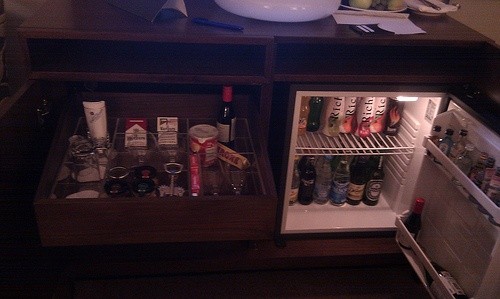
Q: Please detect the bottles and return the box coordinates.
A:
[397,198,424,250]
[421,252,470,299]
[290,155,300,205]
[306,96,323,132]
[216,86,237,149]
[447,129,468,163]
[313,155,332,205]
[362,155,384,206]
[298,96,309,132]
[432,129,454,164]
[427,125,442,157]
[298,156,316,205]
[346,155,367,205]
[452,144,474,186]
[464,152,500,230]
[331,156,350,206]
[321,95,404,137]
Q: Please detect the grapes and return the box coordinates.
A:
[370,0,388,11]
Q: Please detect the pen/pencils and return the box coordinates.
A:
[192,17,244,30]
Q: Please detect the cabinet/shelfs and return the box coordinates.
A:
[0,0,500,299]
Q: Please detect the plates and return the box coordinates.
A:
[339,0,451,17]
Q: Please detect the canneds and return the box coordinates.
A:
[189,124,218,167]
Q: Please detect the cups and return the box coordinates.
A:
[70,133,188,197]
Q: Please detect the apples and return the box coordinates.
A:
[349,0,371,9]
[387,0,403,11]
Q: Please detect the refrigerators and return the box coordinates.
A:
[275,79,500,299]
